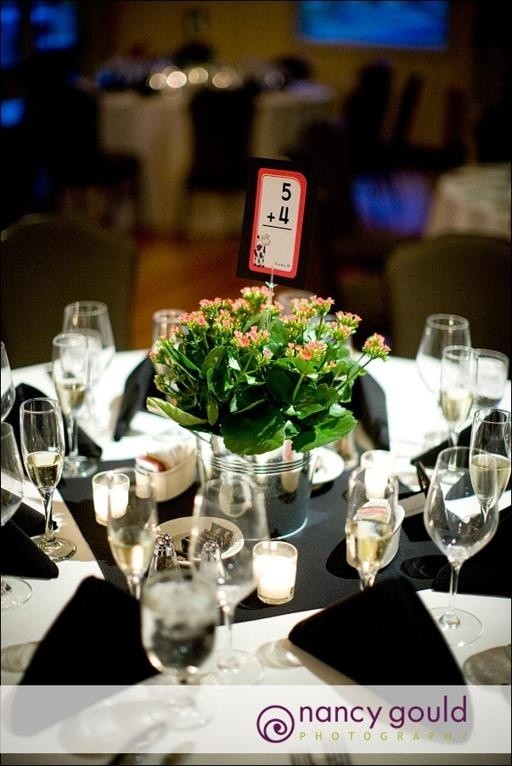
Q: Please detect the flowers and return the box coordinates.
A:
[145,286,391,457]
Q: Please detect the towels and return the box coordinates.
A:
[115,354,166,446]
[411,408,512,470]
[8,575,162,734]
[2,382,104,459]
[287,574,472,743]
[341,362,389,451]
[0,488,60,577]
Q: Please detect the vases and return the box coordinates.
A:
[196,431,315,542]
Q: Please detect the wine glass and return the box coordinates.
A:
[109,465,159,605]
[346,464,405,591]
[417,445,501,651]
[416,311,512,530]
[141,535,218,729]
[187,476,271,687]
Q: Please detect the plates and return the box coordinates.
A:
[157,516,244,568]
[242,440,345,493]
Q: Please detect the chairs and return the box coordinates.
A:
[386,230,511,374]
[42,40,512,244]
[0,211,138,369]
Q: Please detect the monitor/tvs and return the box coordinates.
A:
[296,1,448,56]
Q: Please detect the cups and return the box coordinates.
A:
[253,540,298,607]
[89,471,129,524]
[153,306,187,348]
